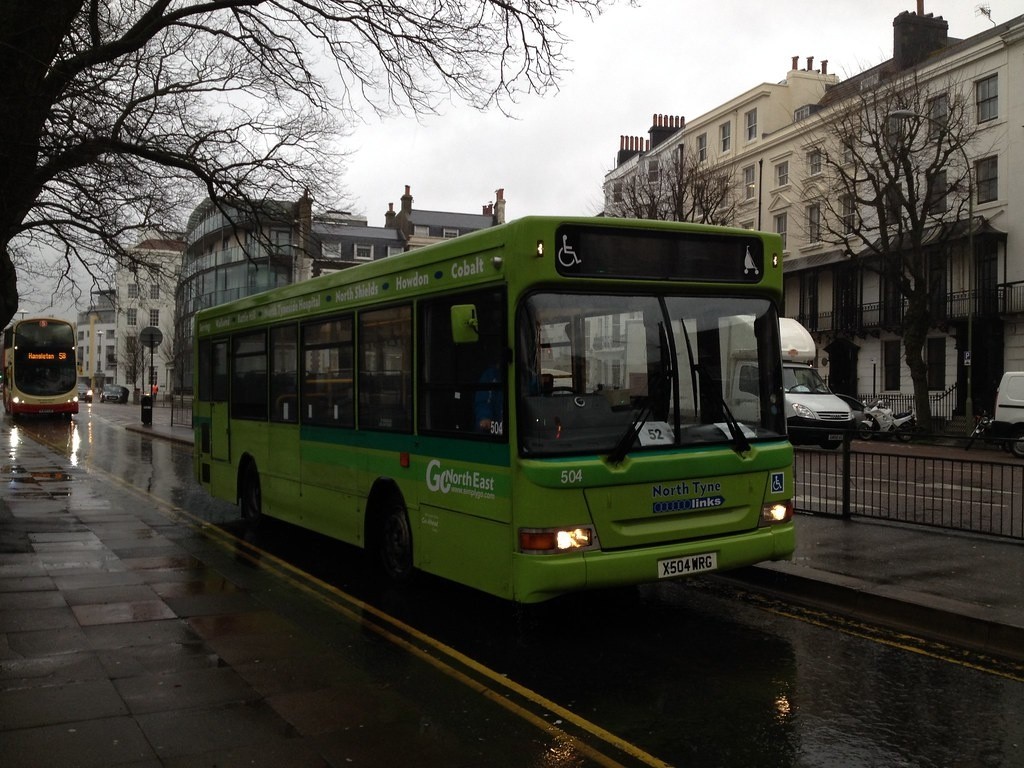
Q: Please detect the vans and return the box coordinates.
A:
[994,371,1024,453]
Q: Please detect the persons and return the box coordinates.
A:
[473,339,544,432]
[152,381,159,402]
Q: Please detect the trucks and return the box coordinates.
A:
[620,314,858,451]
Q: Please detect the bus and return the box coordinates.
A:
[189,215,795,606]
[2,317,79,423]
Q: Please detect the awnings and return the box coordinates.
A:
[783,215,1008,275]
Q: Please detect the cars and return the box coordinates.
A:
[78,382,93,403]
[100,384,129,403]
[835,393,865,429]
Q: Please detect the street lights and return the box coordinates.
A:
[890,110,972,397]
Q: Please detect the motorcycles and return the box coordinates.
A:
[858,401,918,442]
[964,408,1024,459]
